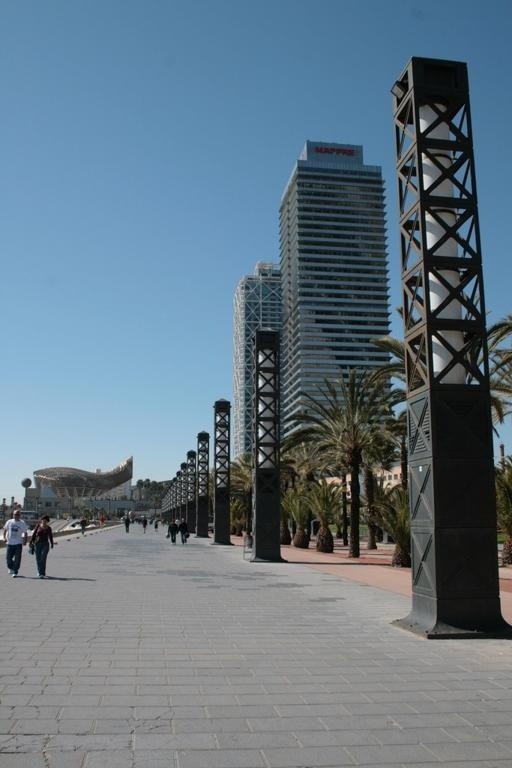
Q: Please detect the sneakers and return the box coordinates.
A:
[8,569,17,577]
[40,575,44,579]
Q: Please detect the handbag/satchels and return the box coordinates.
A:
[166,534,169,538]
[28,543,34,554]
[185,534,189,538]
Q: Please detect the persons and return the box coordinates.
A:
[178,518,188,545]
[142,518,147,534]
[81,516,87,534]
[168,519,179,544]
[29,514,55,579]
[125,517,131,533]
[3,509,29,578]
